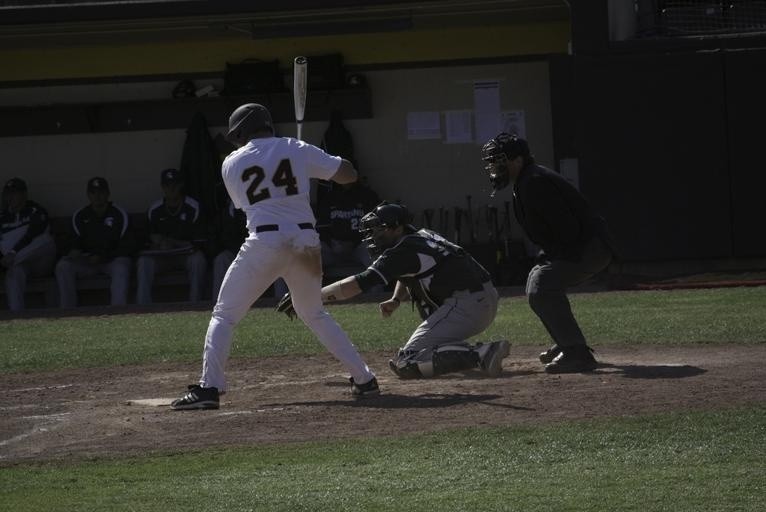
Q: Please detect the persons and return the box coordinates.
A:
[135,167,210,304]
[53,176,130,308]
[0,177,45,311]
[211,195,286,308]
[311,160,382,294]
[276,201,514,379]
[166,102,384,411]
[479,133,616,375]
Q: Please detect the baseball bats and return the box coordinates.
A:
[293,57,307,140]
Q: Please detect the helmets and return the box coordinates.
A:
[479,132,529,194]
[222,102,277,146]
[357,197,412,260]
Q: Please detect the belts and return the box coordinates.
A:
[255,223,314,234]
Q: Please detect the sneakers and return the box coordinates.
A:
[538,343,601,374]
[346,376,382,396]
[479,337,511,378]
[168,383,220,411]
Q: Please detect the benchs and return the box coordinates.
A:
[0,213,201,308]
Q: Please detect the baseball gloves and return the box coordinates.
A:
[274,293,297,318]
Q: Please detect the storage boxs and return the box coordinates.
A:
[302,50,346,91]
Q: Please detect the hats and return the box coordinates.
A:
[2,177,28,193]
[161,168,189,184]
[86,175,109,192]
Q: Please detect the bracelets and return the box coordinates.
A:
[392,297,400,307]
